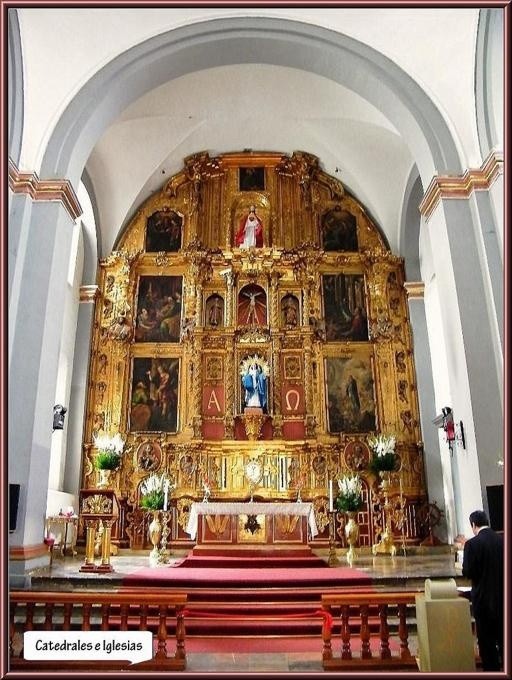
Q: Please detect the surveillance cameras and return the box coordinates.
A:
[431,412,451,426]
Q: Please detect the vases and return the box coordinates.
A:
[373,471,400,555]
[97,467,111,489]
[149,510,162,559]
[345,512,359,560]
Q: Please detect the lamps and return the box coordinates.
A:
[53,404,67,429]
[446,420,466,453]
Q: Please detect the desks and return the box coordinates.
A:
[46,516,79,557]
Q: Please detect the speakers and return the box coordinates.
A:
[487,484,504,534]
[8,483,20,534]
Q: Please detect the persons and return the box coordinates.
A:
[341,307,368,341]
[138,445,159,471]
[462,510,504,672]
[326,204,354,236]
[146,364,177,422]
[237,206,262,249]
[209,298,221,324]
[345,375,360,409]
[242,363,268,409]
[243,290,262,324]
[346,443,367,470]
[282,297,296,324]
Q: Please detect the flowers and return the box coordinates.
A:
[367,434,397,473]
[140,476,168,509]
[92,433,125,469]
[337,476,364,512]
